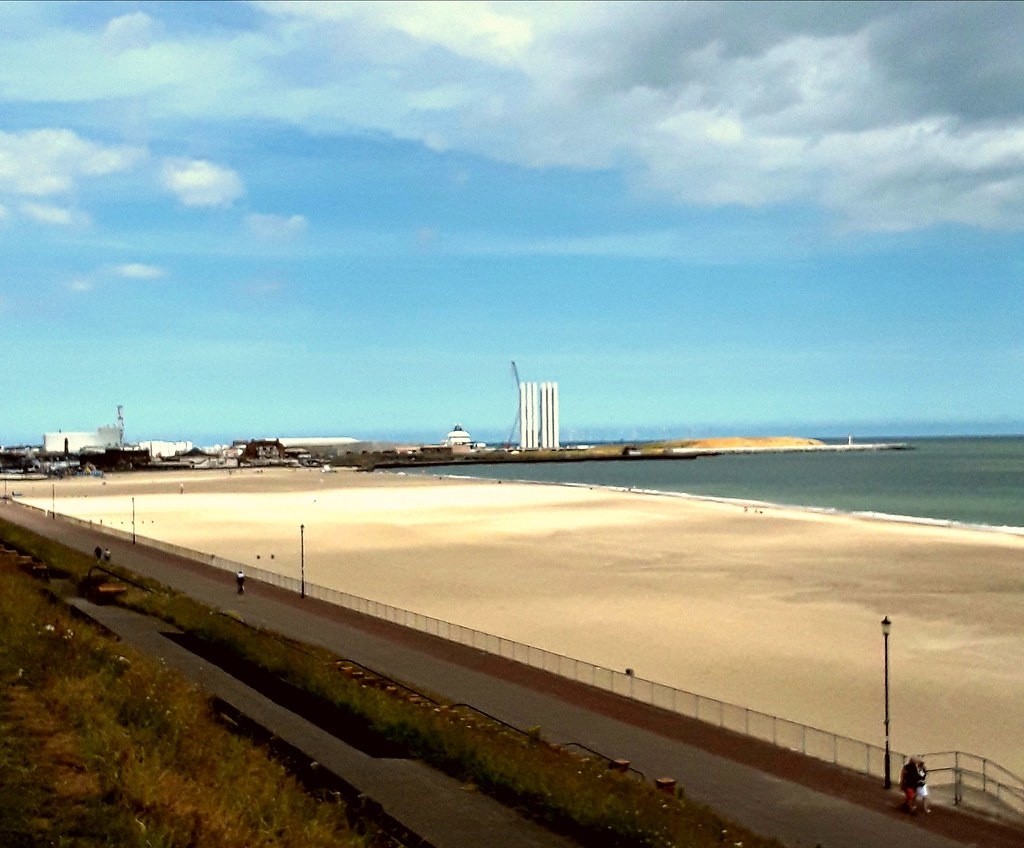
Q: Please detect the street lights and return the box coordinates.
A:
[300,524,305,598]
[53,484,54,519]
[881,615,893,790]
[132,497,135,544]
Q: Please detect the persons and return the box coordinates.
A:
[236,571,245,594]
[94,545,102,559]
[915,760,932,814]
[898,755,920,815]
[104,548,110,560]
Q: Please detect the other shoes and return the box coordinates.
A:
[927,810,932,815]
